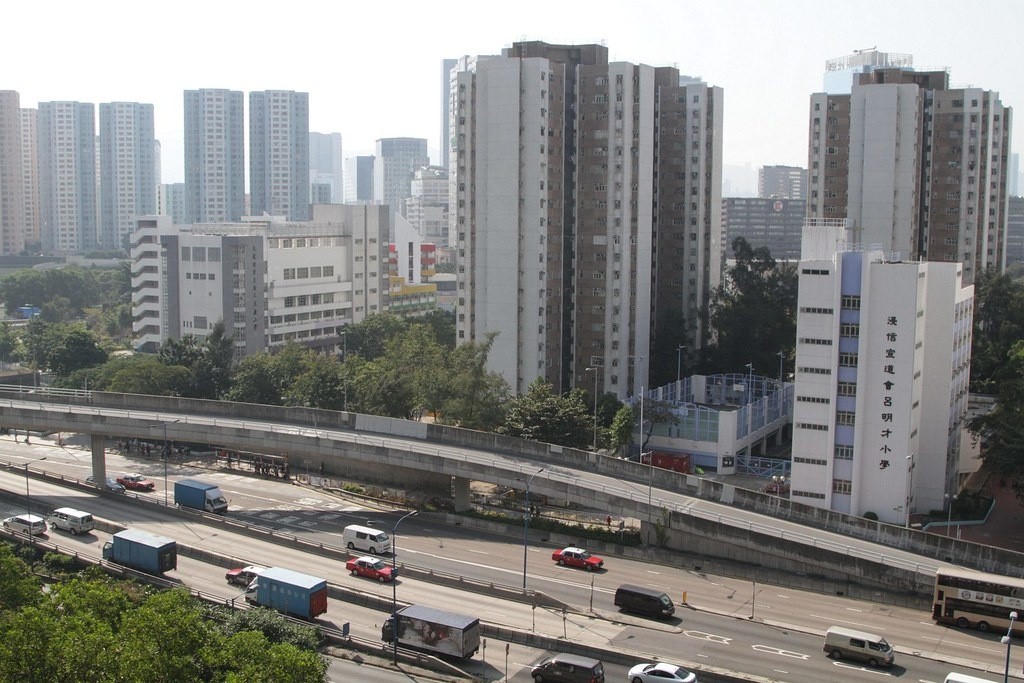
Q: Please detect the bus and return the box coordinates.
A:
[931,566,1024,636]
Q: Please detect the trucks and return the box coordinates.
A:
[381,603,480,662]
[173,479,232,515]
[103,528,177,576]
[245,566,328,621]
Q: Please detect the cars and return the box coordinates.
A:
[628,663,698,683]
[86,473,155,494]
[552,547,604,571]
[346,555,394,582]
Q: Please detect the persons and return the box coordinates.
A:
[529,505,540,517]
[117,439,192,461]
[481,494,487,511]
[220,451,291,480]
[619,521,626,531]
[321,462,325,477]
[423,623,436,646]
[606,515,612,528]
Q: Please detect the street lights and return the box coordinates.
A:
[340,331,347,412]
[945,494,957,536]
[745,364,757,466]
[772,475,786,495]
[777,352,785,417]
[586,367,598,451]
[26,303,37,396]
[676,345,686,401]
[6,456,47,573]
[150,419,180,507]
[367,510,419,664]
[515,468,545,594]
[906,455,913,527]
[642,452,652,545]
[1001,611,1018,683]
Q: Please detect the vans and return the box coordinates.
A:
[3,507,94,536]
[614,583,675,618]
[343,524,390,554]
[823,626,894,666]
[530,652,605,683]
[944,672,998,683]
